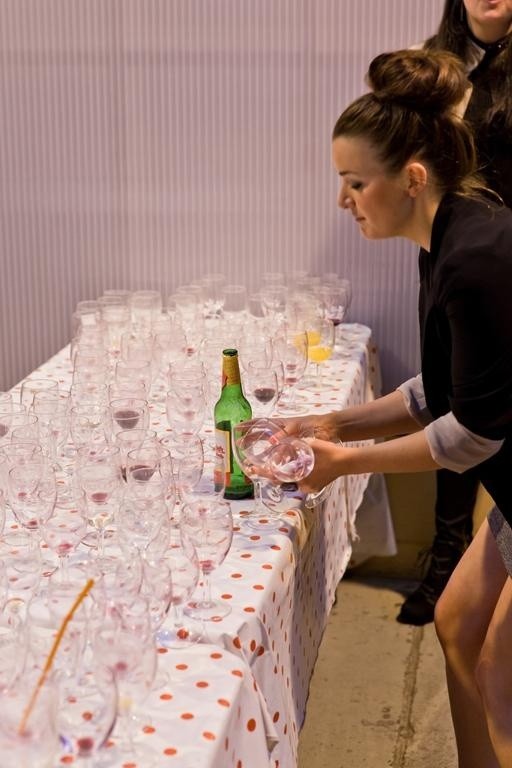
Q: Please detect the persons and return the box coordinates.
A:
[236,46,511,768]
[395,1,511,627]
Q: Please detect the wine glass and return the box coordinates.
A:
[0,263,352,768]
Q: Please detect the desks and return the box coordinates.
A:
[0,320,399,768]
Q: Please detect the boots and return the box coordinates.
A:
[396,493,478,626]
